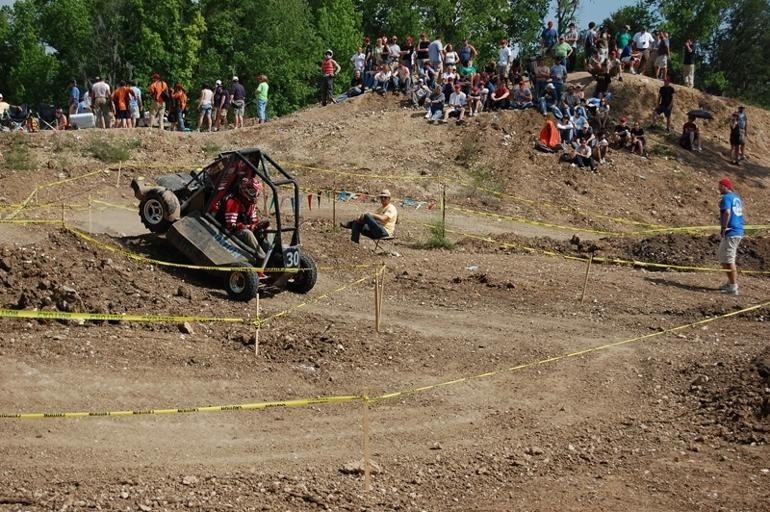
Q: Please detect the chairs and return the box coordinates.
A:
[373,216,401,257]
[4,102,32,131]
[36,103,58,132]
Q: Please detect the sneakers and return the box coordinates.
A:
[455,119,465,126]
[438,118,449,124]
[721,284,739,295]
[429,118,436,125]
[203,128,212,132]
[468,111,473,117]
[473,111,479,118]
[194,128,202,132]
[340,220,353,229]
[718,282,734,290]
[425,111,433,119]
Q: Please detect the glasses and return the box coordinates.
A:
[379,195,389,199]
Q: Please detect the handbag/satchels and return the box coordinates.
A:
[161,90,172,103]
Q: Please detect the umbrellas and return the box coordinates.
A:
[686,108,713,129]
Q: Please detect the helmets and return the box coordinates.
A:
[236,177,263,204]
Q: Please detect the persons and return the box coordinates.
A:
[252,75,269,125]
[229,76,246,128]
[149,72,168,130]
[730,113,740,165]
[321,49,341,106]
[717,177,743,296]
[196,79,230,132]
[341,188,397,244]
[736,104,747,161]
[170,84,190,131]
[350,21,676,171]
[228,179,271,258]
[683,36,696,89]
[0,75,143,131]
[680,116,702,152]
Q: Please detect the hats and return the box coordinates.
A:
[500,38,508,44]
[576,108,583,114]
[215,79,222,86]
[563,114,570,118]
[150,73,161,80]
[375,189,392,197]
[546,83,556,91]
[231,75,240,82]
[717,176,735,193]
[325,49,334,55]
[453,83,462,89]
[471,86,481,93]
[620,115,629,123]
[587,126,594,133]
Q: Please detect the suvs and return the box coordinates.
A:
[131,148,317,302]
